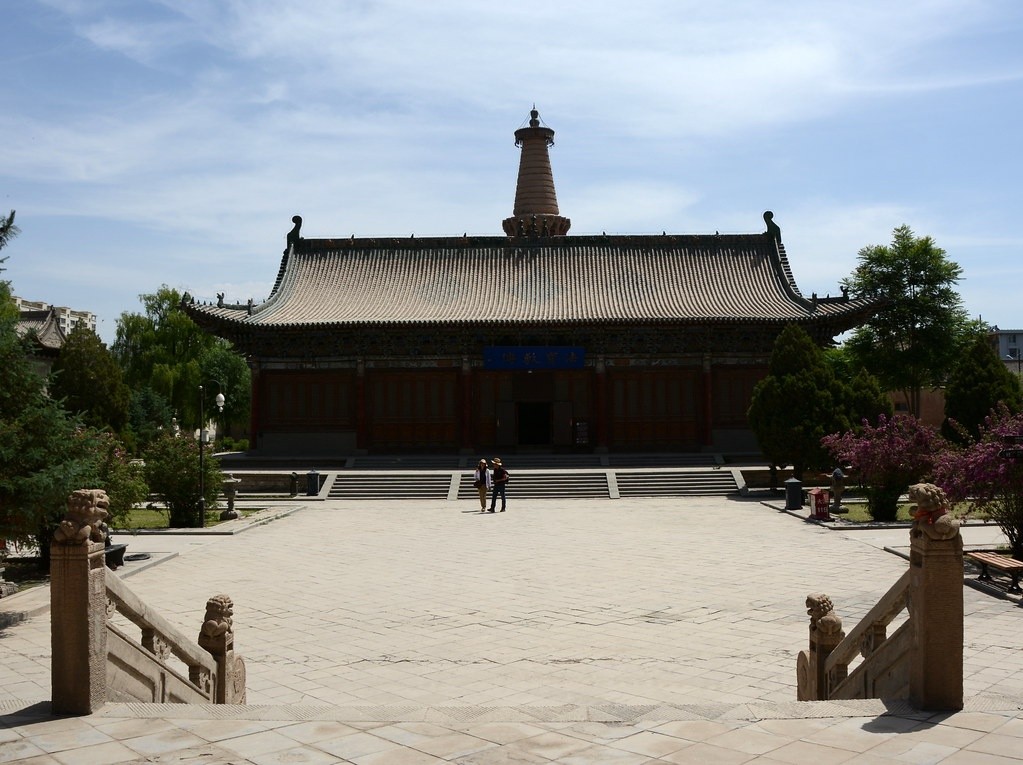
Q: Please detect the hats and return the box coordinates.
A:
[491,458,502,465]
[479,459,488,467]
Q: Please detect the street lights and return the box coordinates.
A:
[198,378,225,528]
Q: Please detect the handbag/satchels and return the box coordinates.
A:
[505,471,509,483]
[474,480,484,488]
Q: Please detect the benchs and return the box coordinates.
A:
[105,544,129,571]
[967,552,1023,594]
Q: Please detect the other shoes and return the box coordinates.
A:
[488,508,495,513]
[500,508,505,512]
[481,507,485,511]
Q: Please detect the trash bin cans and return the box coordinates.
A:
[307,468,319,496]
[784,474,803,510]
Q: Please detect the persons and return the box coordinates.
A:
[486,458,507,512]
[473,459,490,512]
